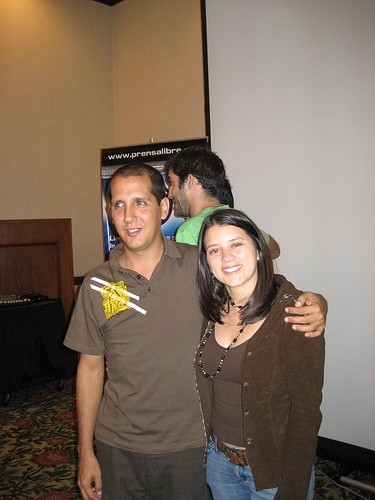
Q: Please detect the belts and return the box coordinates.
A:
[217,440,248,467]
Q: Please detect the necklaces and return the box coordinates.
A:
[198,296,247,377]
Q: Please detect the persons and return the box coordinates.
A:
[194,209,325,500]
[64,162,328,500]
[163,149,280,260]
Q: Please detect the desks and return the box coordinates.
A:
[0,295,78,406]
